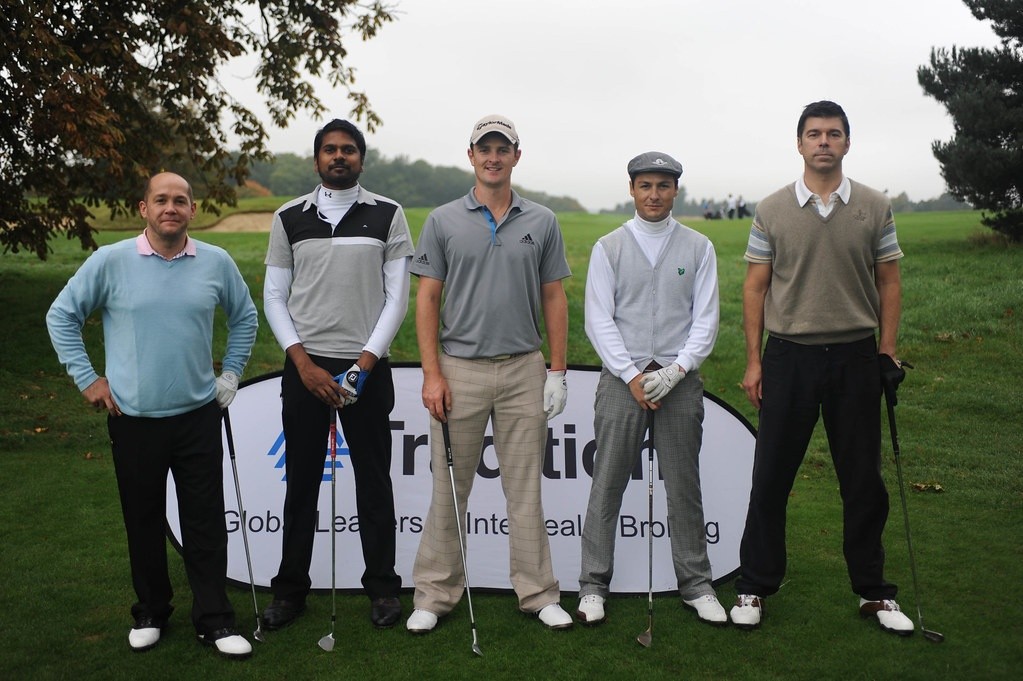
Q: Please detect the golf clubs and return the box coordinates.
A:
[224,403,267,645]
[883,385,945,643]
[317,404,339,653]
[439,399,485,657]
[636,408,657,649]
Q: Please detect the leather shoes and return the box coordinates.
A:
[731,592,763,626]
[199,624,252,656]
[128,616,163,649]
[576,594,606,624]
[532,602,573,628]
[408,609,438,635]
[859,595,914,635]
[682,590,727,625]
[371,593,400,628]
[262,593,306,630]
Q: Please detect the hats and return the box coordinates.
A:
[469,116,521,152]
[628,153,682,181]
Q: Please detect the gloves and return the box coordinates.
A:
[872,351,907,406]
[332,363,370,408]
[214,367,239,410]
[638,363,685,403]
[543,367,571,421]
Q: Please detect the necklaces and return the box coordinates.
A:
[501,200,513,216]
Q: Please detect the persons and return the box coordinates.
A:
[46,172,257,656]
[701,194,751,220]
[257,119,404,628]
[575,151,729,625]
[406,114,572,633]
[730,101,915,635]
